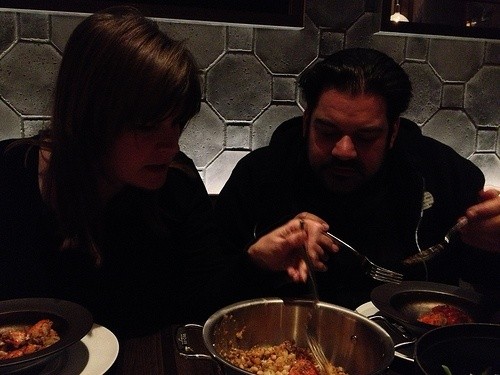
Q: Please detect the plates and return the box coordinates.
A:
[9,323,119,375]
[355,301,415,362]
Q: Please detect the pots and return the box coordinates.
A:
[168,297,417,375]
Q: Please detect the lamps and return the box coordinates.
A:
[390,0,409,23]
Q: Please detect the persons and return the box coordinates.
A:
[216,48,500,307]
[0,5,324,323]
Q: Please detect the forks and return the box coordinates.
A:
[325,231,403,284]
[405,215,468,263]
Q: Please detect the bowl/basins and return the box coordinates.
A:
[415,323,500,375]
[370,281,494,333]
[0,299,94,375]
